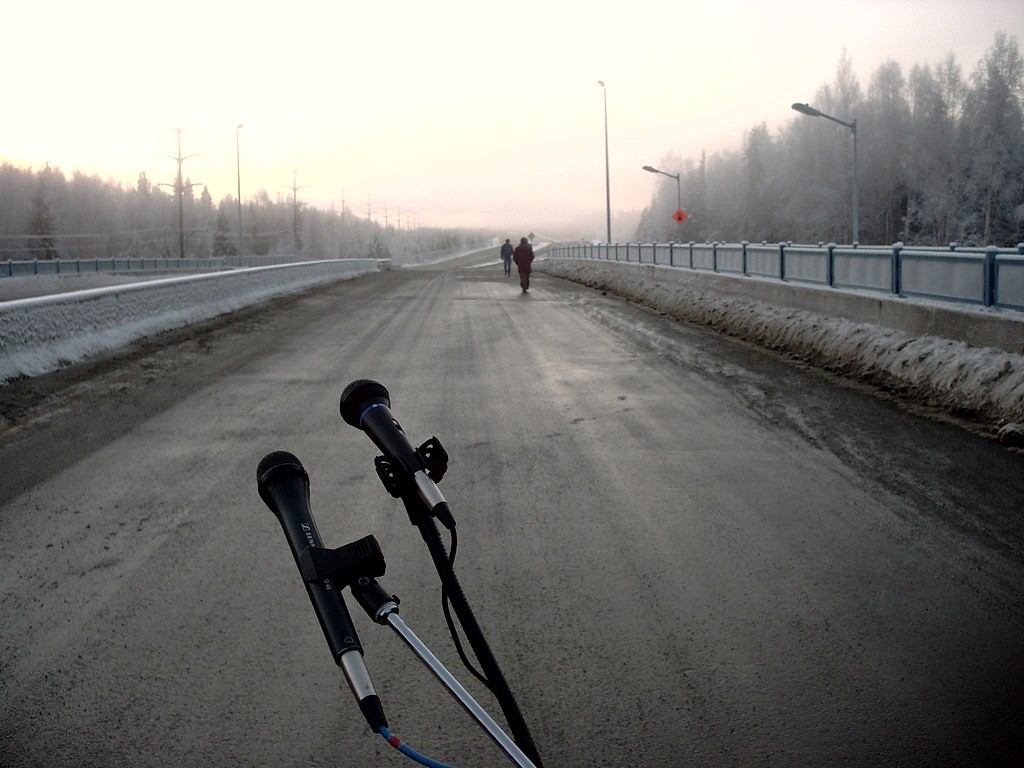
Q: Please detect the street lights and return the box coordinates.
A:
[641,165,683,242]
[598,80,613,243]
[792,101,860,245]
[236,124,244,265]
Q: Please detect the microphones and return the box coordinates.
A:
[258,450,388,733]
[341,379,457,531]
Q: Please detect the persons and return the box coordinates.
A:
[513,238,535,295]
[501,239,514,277]
